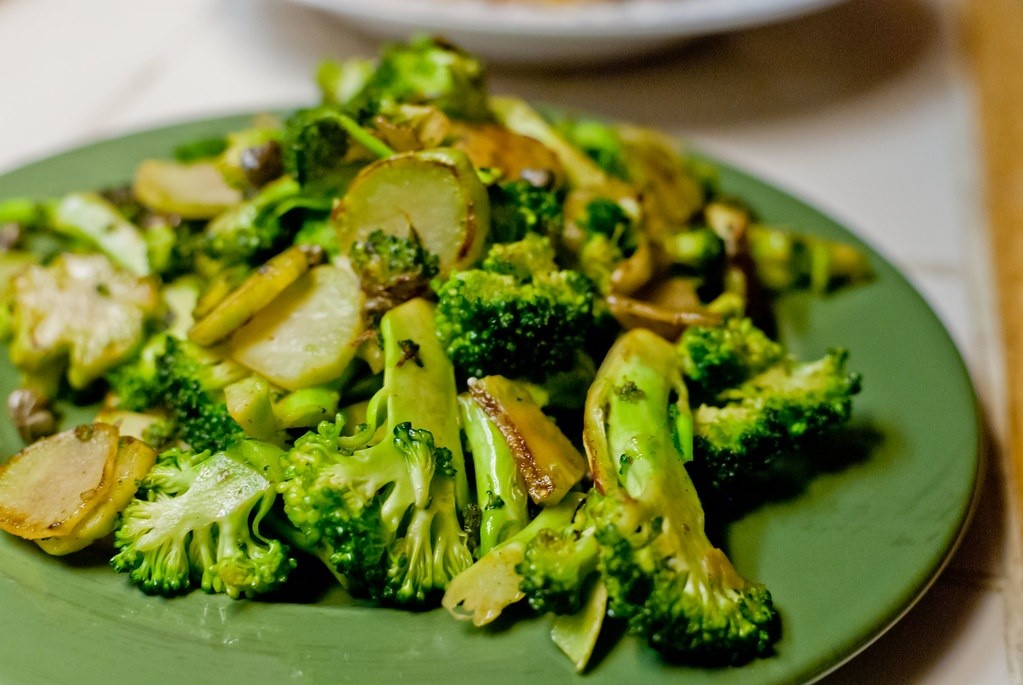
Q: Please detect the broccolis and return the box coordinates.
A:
[2,34,863,662]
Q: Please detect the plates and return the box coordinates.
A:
[1,106,982,685]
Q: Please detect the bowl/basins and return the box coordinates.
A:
[301,1,844,68]
